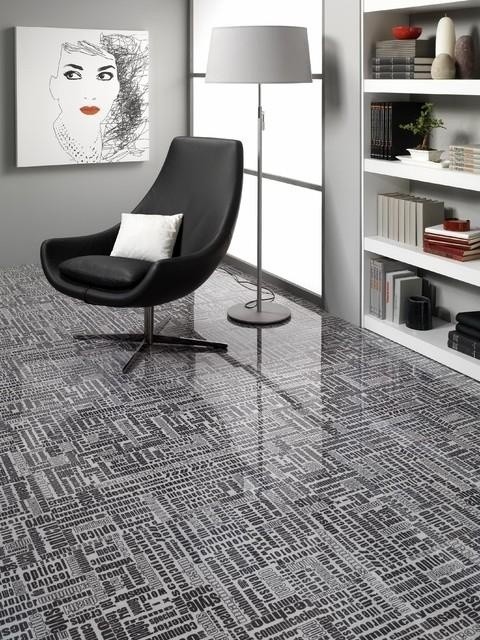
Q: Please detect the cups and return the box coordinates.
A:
[407,294,434,331]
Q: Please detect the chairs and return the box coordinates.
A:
[40,133,247,375]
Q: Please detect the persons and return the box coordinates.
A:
[49,33,145,164]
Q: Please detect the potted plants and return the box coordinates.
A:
[397,101,451,164]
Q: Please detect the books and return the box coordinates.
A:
[370,36,436,80]
[370,100,479,175]
[366,191,479,360]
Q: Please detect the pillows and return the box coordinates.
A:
[102,211,185,263]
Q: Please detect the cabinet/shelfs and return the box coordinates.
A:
[359,3,480,385]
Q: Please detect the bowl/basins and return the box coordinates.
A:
[391,27,422,39]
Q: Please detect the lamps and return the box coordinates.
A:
[203,23,317,329]
